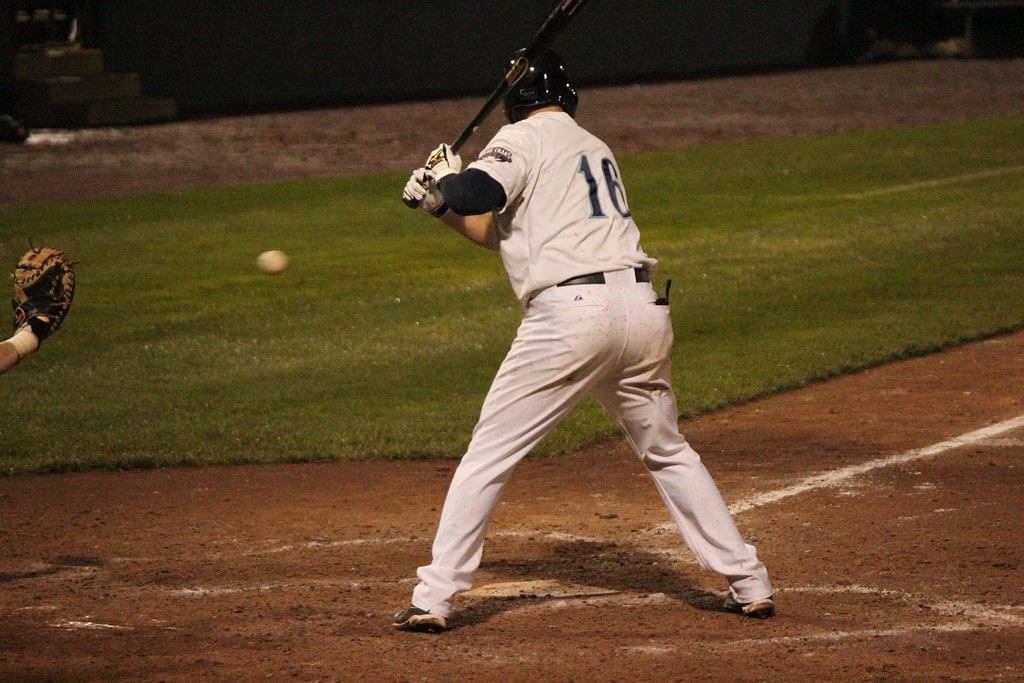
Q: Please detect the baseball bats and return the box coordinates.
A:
[402,0,590,209]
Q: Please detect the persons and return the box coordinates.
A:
[389,45,777,634]
[0,314,50,374]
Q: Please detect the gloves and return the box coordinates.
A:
[402,168,450,220]
[424,143,462,181]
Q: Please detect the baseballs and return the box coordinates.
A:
[256,249,288,273]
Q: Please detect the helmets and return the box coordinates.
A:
[502,48,578,121]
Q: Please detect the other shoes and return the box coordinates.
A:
[393,605,448,629]
[726,596,774,615]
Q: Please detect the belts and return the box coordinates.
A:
[557,268,650,286]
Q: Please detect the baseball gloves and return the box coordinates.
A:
[12,247,76,344]
